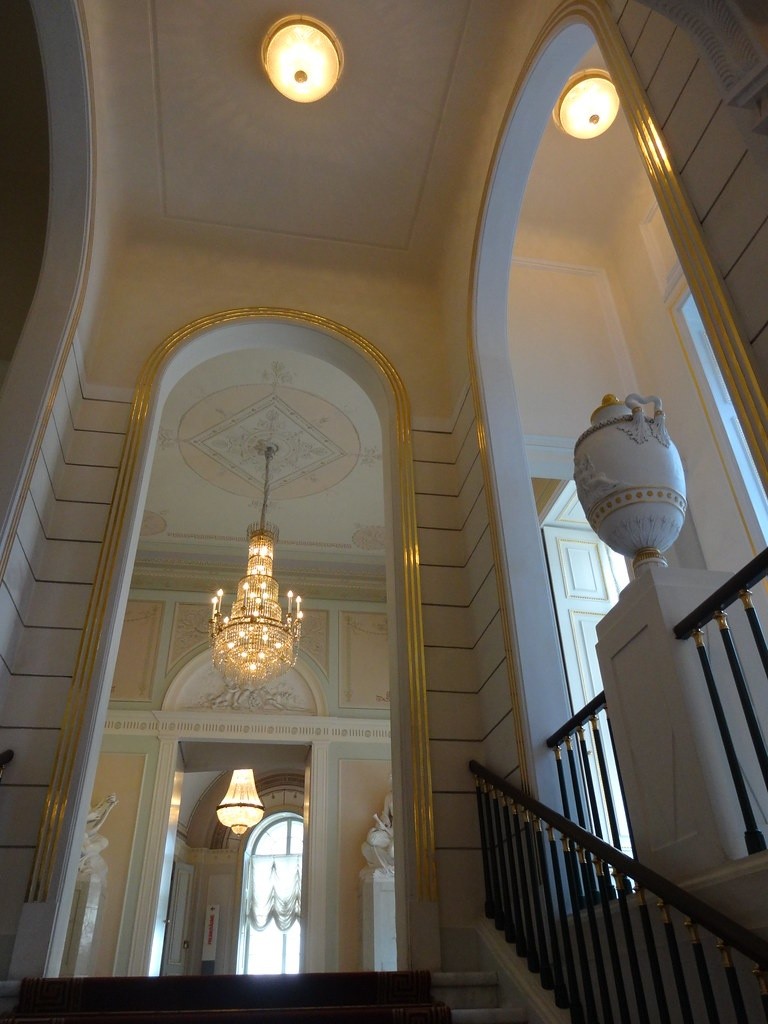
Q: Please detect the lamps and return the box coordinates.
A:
[216,769,266,837]
[209,439,305,680]
[553,67,620,139]
[261,13,345,103]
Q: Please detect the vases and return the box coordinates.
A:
[573,392,689,575]
